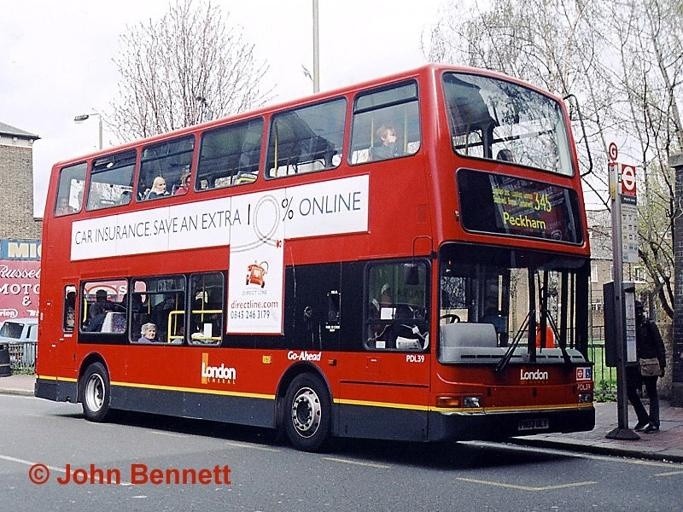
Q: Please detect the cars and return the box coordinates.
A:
[0,318,37,367]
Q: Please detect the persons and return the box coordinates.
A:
[626,297,667,432]
[88,290,115,320]
[174,173,191,195]
[365,123,407,163]
[137,321,158,343]
[58,196,74,214]
[66,291,75,327]
[145,176,169,199]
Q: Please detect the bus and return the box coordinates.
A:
[30,65,595,456]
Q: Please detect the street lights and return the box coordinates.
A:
[71,111,103,151]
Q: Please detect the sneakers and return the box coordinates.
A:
[634,421,648,429]
[645,425,658,434]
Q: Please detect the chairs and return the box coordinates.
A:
[98,199,116,208]
[234,168,259,185]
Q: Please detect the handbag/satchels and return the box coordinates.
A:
[639,358,662,376]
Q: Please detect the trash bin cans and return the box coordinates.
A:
[0,342,11,377]
